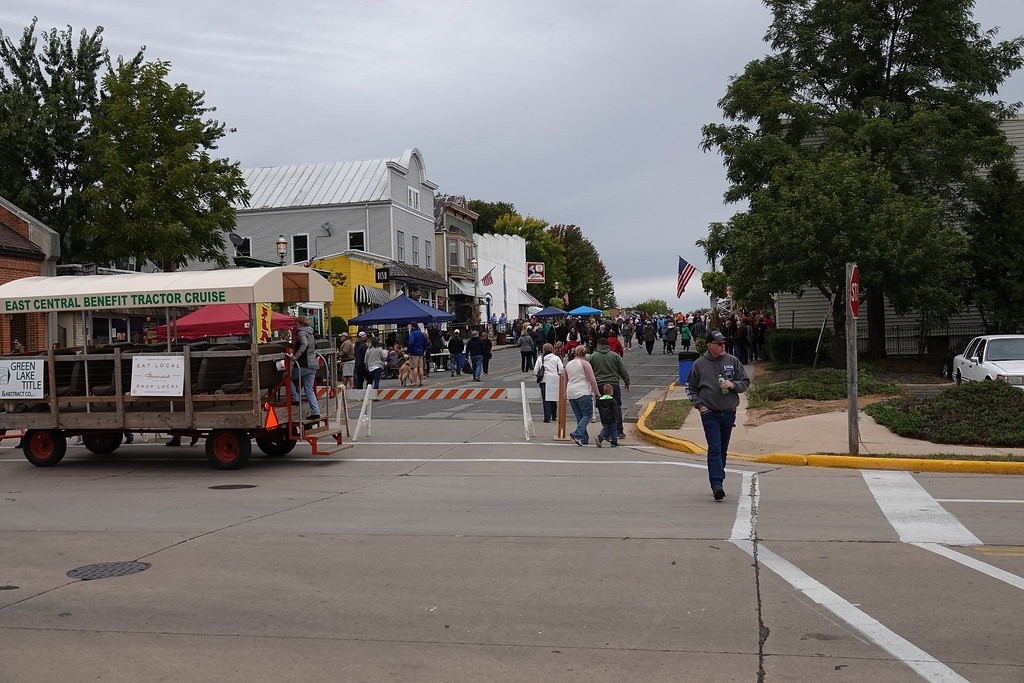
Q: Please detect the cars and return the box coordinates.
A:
[942,337,975,379]
[951,334,1024,390]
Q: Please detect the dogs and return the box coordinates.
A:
[399,361,414,387]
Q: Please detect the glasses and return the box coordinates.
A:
[711,341,726,346]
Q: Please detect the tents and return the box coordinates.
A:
[569,305,600,316]
[532,305,568,325]
[348,294,456,340]
[158,304,296,356]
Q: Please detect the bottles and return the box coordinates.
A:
[718,374,729,395]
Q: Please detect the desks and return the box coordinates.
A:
[431,353,451,372]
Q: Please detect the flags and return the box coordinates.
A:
[676,257,696,299]
[482,272,493,286]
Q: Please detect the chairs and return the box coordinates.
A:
[38,340,330,409]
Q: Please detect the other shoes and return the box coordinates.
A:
[476,377,480,382]
[190,437,198,446]
[291,401,299,405]
[571,433,625,448]
[75,440,84,445]
[166,438,181,446]
[125,434,133,444]
[306,415,320,419]
[15,443,22,448]
[714,488,725,503]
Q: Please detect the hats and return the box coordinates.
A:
[472,331,480,335]
[339,332,348,336]
[358,331,366,338]
[454,329,459,334]
[12,339,22,344]
[706,331,726,343]
[670,315,674,318]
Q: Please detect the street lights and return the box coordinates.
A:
[589,287,594,308]
[554,281,559,298]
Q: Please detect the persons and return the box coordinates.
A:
[109,336,199,446]
[685,331,751,503]
[9,338,29,448]
[488,309,774,372]
[533,338,631,448]
[284,317,321,419]
[336,321,493,402]
[52,342,60,350]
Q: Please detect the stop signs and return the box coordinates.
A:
[850,265,860,319]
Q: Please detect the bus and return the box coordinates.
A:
[0,264,354,471]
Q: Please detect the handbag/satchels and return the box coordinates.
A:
[746,336,754,347]
[462,361,473,374]
[536,365,545,383]
[489,353,492,359]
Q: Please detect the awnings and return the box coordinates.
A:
[354,284,391,304]
[518,288,543,306]
